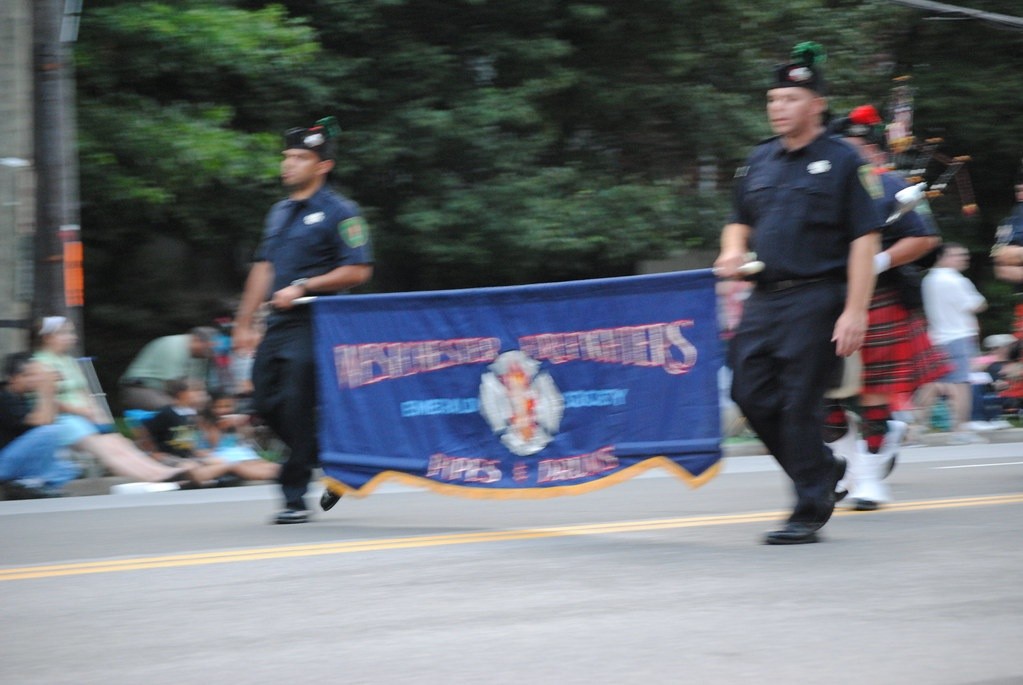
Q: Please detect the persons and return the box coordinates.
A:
[0,103,1023,523]
[711,44,883,544]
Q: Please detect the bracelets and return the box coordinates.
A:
[874,252,892,273]
[293,277,312,297]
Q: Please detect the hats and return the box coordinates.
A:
[826,105,884,142]
[765,41,827,92]
[284,124,331,161]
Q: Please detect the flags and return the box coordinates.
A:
[304,274,723,499]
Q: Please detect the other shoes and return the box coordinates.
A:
[784,456,847,533]
[824,409,864,501]
[276,507,308,522]
[767,530,815,543]
[846,420,907,509]
[321,490,341,511]
